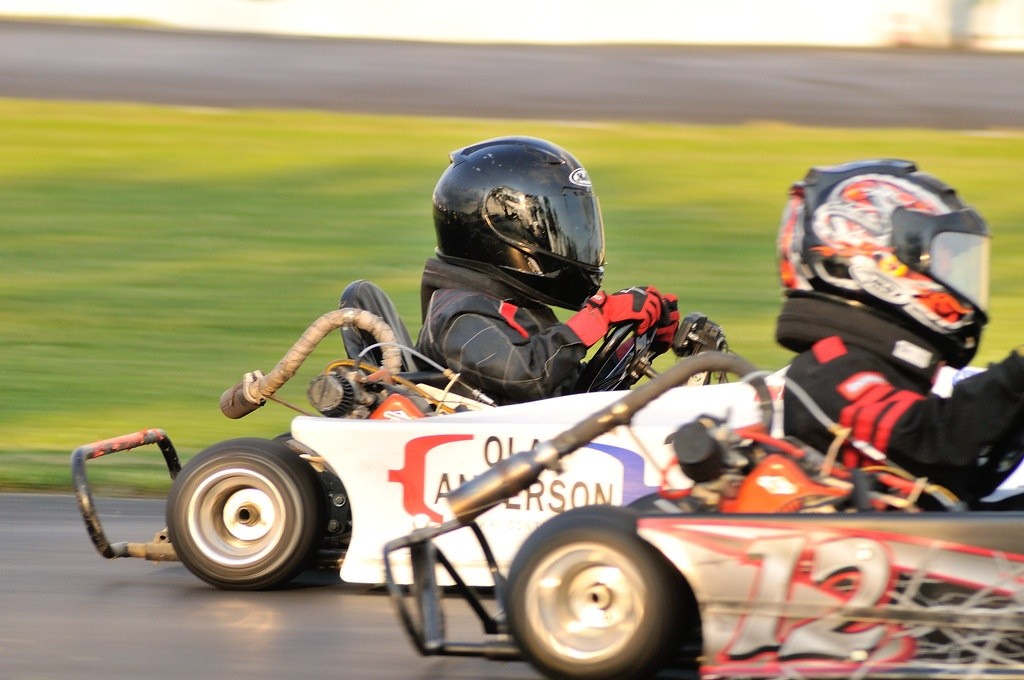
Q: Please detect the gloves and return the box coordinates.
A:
[616,295,679,363]
[566,286,663,346]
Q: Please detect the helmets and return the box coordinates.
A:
[431,137,605,313]
[776,160,990,386]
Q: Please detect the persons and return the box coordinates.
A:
[774,158,1024,510]
[410,136,680,405]
